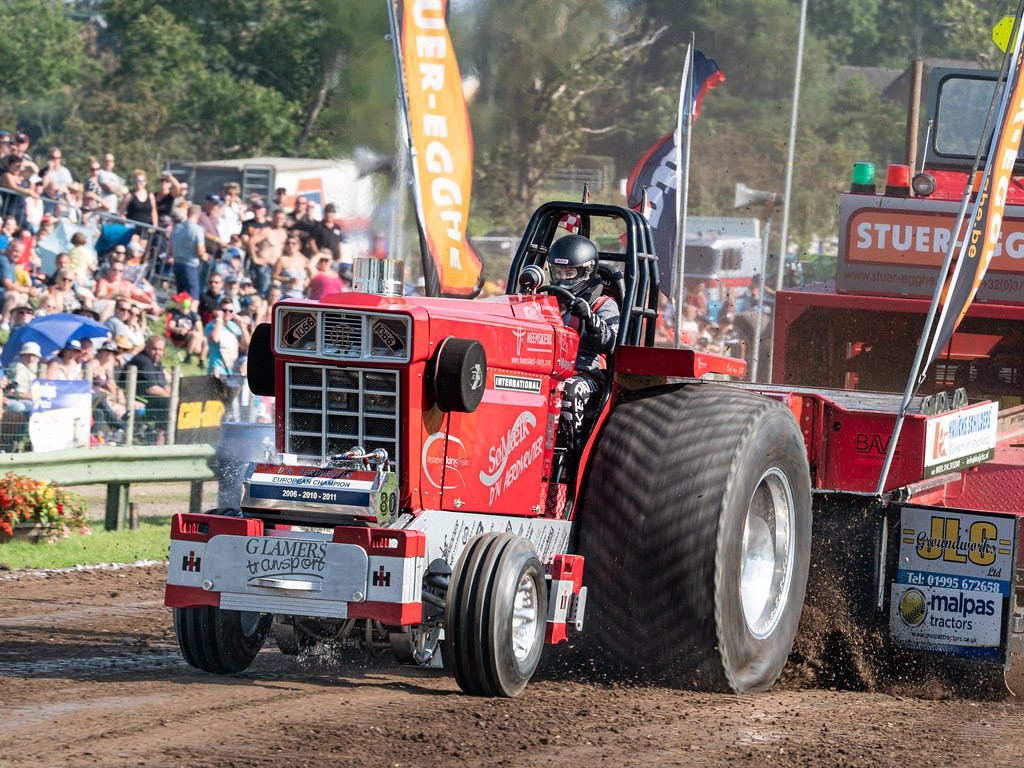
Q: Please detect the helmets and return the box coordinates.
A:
[546,235,599,295]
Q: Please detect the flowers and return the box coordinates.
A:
[0,470,94,544]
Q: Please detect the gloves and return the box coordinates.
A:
[568,297,591,320]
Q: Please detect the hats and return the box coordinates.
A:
[224,276,252,285]
[72,305,100,321]
[66,338,81,353]
[159,215,174,227]
[205,194,226,206]
[102,152,115,162]
[172,291,194,303]
[251,200,266,208]
[96,340,121,355]
[324,203,337,212]
[159,170,172,183]
[19,342,42,358]
[9,302,33,315]
[14,133,30,143]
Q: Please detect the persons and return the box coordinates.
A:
[0,133,736,438]
[545,234,621,472]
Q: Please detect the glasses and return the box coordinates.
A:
[17,310,33,314]
[221,309,233,313]
[208,281,219,284]
[112,251,126,272]
[120,307,140,318]
[89,168,100,171]
[297,201,314,208]
[62,278,72,282]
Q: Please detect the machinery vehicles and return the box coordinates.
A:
[166,181,813,696]
[751,0,1024,693]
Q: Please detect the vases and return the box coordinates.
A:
[0,522,66,544]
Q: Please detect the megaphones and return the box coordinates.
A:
[734,183,784,211]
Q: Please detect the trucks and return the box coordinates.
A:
[682,216,763,321]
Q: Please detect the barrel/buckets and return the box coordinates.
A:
[217,422,275,509]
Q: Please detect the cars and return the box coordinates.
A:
[471,235,522,257]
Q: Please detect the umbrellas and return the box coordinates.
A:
[51,312,110,341]
[1,314,84,369]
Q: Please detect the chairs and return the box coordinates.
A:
[198,303,217,325]
[164,312,189,362]
[597,263,625,317]
